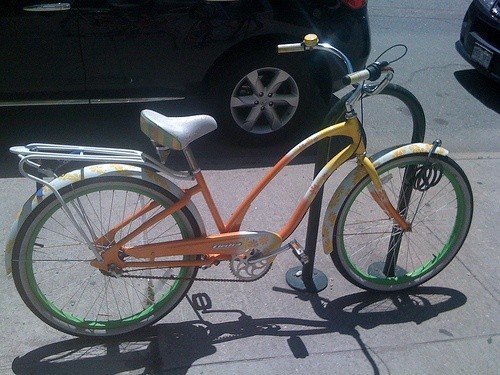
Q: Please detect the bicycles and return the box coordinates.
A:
[0,34,475,344]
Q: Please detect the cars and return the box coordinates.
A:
[454,0,500,84]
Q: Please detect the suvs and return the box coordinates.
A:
[0,2,371,139]
